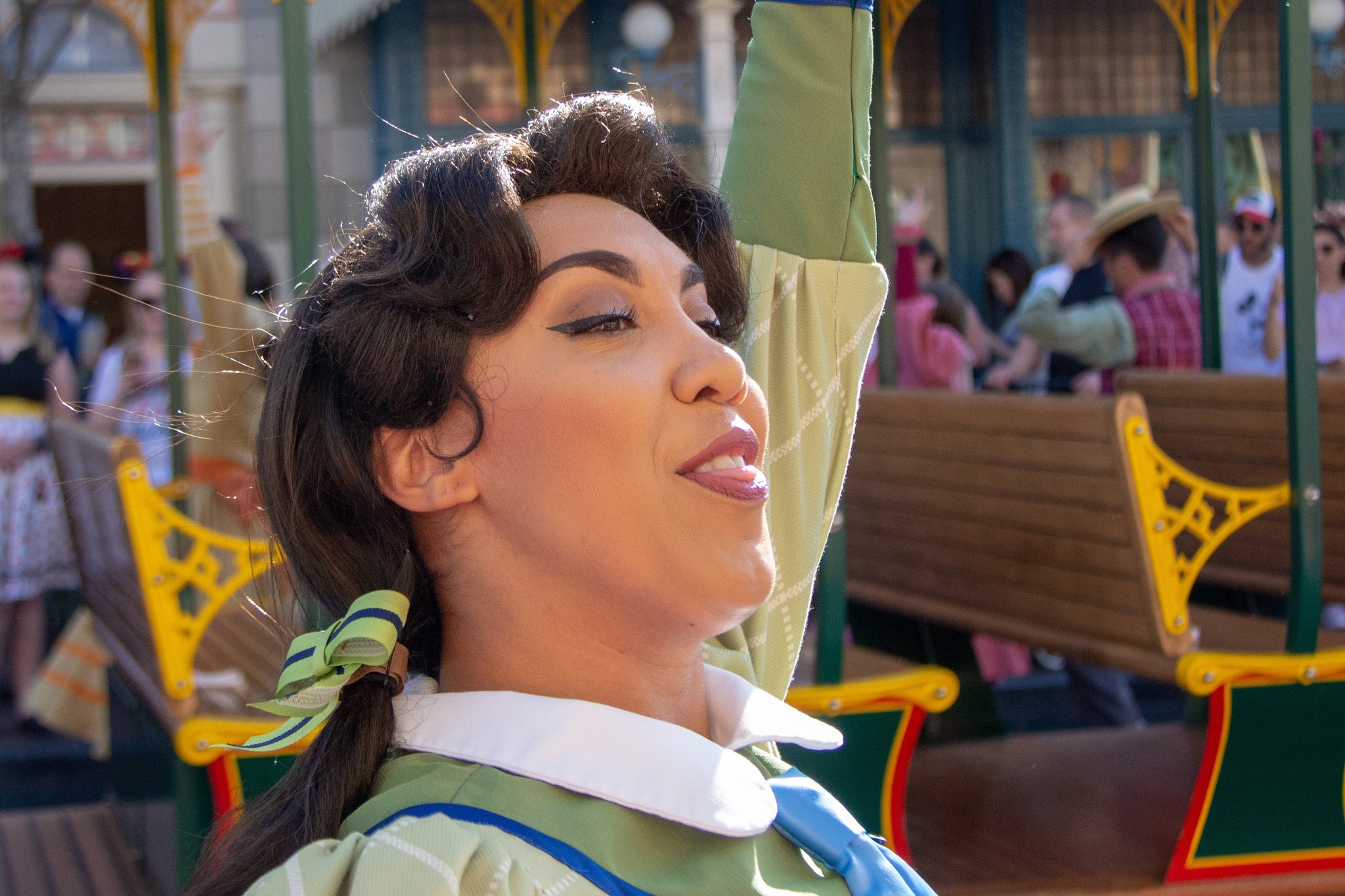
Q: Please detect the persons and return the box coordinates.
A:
[839,186,1345,746]
[193,89,933,896]
[1,221,283,729]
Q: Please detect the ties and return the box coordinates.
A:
[742,745,942,895]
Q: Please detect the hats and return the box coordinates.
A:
[1232,191,1277,224]
[1085,187,1181,250]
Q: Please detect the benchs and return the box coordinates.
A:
[784,640,961,865]
[845,392,1345,885]
[1116,370,1345,605]
[47,408,326,865]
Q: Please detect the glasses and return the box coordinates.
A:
[1234,221,1266,233]
